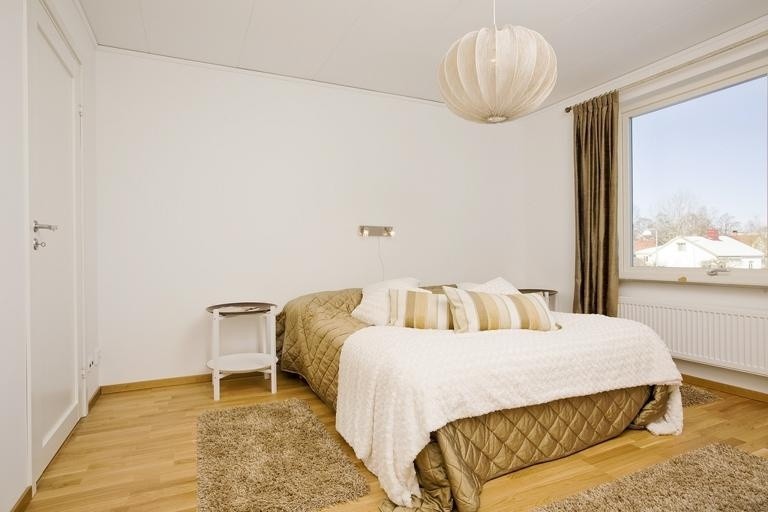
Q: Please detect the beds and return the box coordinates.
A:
[277,283,685,511]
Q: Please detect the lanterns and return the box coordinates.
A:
[437,22,559,127]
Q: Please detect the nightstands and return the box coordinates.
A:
[206,301,279,400]
[517,287,560,309]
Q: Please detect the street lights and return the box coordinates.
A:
[648,228,658,267]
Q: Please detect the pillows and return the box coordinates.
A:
[386,289,455,332]
[443,286,563,332]
[454,276,524,295]
[349,286,432,328]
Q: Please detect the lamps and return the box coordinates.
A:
[359,223,396,239]
[438,0,560,124]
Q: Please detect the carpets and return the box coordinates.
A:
[527,438,766,512]
[681,382,717,407]
[194,396,371,511]
